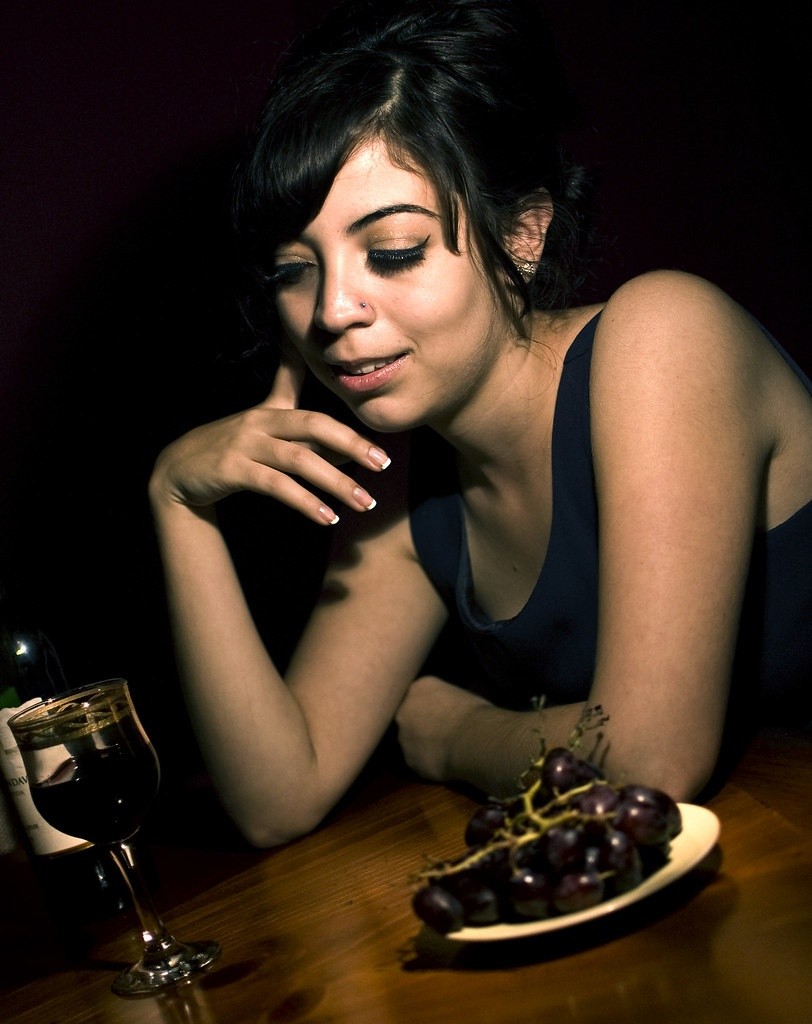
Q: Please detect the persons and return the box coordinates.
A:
[145,0,812,848]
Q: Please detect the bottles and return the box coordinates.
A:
[0,608,144,930]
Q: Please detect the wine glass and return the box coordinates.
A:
[8,676,222,999]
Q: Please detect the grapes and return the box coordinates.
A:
[409,747,682,934]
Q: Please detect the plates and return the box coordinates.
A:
[444,803,722,942]
